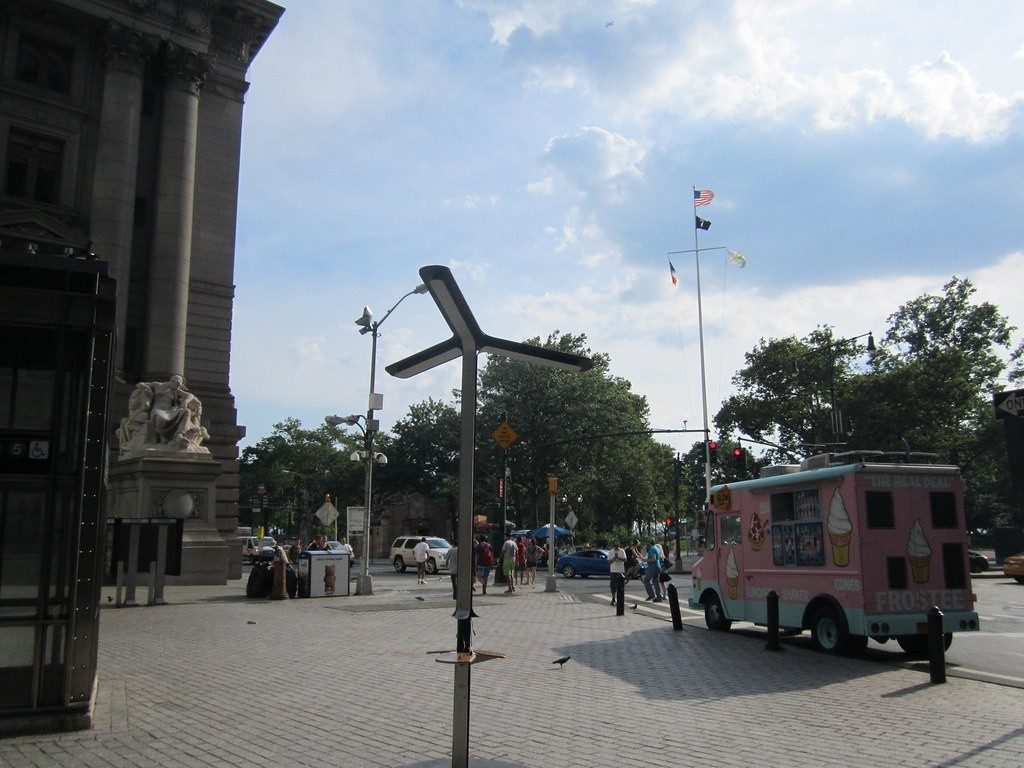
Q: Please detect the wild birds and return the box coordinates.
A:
[552,656,572,669]
[628,603,638,611]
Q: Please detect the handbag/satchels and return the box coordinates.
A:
[660,572,671,583]
[663,557,673,570]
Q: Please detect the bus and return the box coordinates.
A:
[687,437,982,662]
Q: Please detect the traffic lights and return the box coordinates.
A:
[678,461,688,478]
[733,447,748,473]
[696,443,707,464]
[707,441,718,463]
[665,517,673,527]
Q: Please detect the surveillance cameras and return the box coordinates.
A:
[350,452,359,464]
[379,455,388,466]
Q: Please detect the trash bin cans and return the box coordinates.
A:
[298,551,349,597]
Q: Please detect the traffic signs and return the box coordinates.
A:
[992,388,1024,419]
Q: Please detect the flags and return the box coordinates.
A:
[728,250,744,268]
[694,190,714,207]
[696,215,711,230]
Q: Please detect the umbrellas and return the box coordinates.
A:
[531,524,570,537]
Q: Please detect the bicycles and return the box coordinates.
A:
[623,557,654,586]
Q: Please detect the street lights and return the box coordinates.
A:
[324,282,432,600]
[789,328,877,460]
[652,503,659,538]
[283,470,306,542]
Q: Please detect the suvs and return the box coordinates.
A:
[390,536,454,575]
[239,536,277,565]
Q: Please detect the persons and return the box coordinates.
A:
[608,544,627,605]
[500,533,544,593]
[337,537,353,556]
[136,375,203,446]
[308,535,332,550]
[289,538,305,563]
[642,538,671,602]
[625,541,644,576]
[446,540,457,599]
[412,538,430,584]
[473,535,493,594]
[672,537,676,556]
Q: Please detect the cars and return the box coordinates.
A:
[1003,553,1024,583]
[967,550,989,574]
[557,549,612,579]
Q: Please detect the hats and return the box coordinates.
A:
[613,540,620,546]
[648,537,656,544]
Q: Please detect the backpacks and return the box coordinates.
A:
[480,543,494,564]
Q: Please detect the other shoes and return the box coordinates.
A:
[611,599,616,606]
[663,596,667,601]
[421,581,428,584]
[512,588,516,592]
[504,590,513,594]
[646,595,654,602]
[653,597,663,603]
[418,580,421,583]
